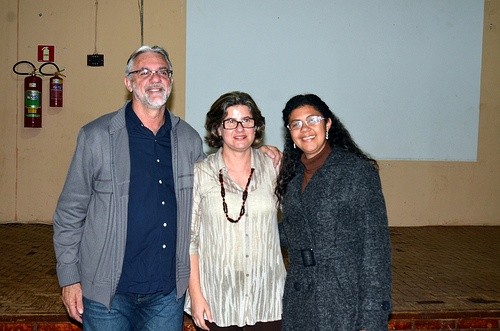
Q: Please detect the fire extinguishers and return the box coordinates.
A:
[24,67,44,128]
[50,68,67,107]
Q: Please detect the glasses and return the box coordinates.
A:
[129,68,172,78]
[221,117,255,130]
[287,115,326,130]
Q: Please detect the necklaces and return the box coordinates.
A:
[217,168,255,224]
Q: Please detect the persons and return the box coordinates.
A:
[269,93,392,331]
[184,91,287,331]
[51,45,283,331]
